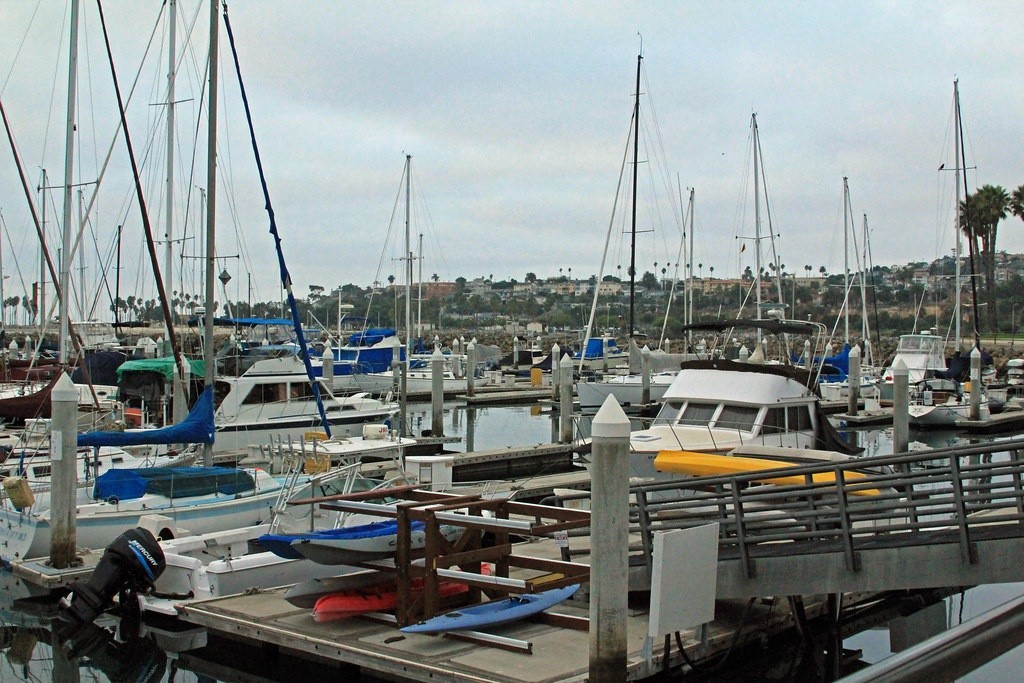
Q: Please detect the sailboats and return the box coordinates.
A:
[572,30,1008,428]
[350,150,490,397]
[0,0,426,558]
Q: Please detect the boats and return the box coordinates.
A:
[572,302,903,506]
[569,334,629,373]
[398,583,581,635]
[653,449,880,499]
[61,435,494,652]
[295,328,451,395]
